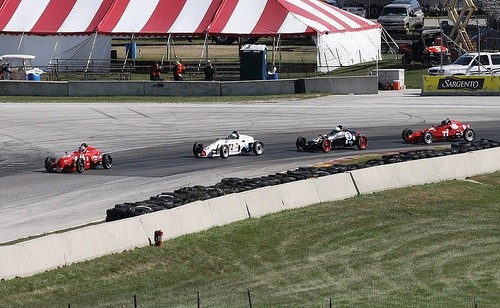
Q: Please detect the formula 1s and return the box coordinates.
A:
[296,124,368,153]
[402,118,475,145]
[45,142,113,173]
[193,131,264,159]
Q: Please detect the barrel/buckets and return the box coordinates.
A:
[393,79,400,90]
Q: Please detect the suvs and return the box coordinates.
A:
[377,0,425,36]
[428,52,500,76]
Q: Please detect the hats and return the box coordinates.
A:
[175,61,180,65]
[207,60,211,63]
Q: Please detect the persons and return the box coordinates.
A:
[203,59,218,81]
[147,62,163,81]
[80,142,88,151]
[414,30,444,50]
[336,125,344,132]
[171,59,185,81]
[232,131,239,139]
[445,117,449,124]
